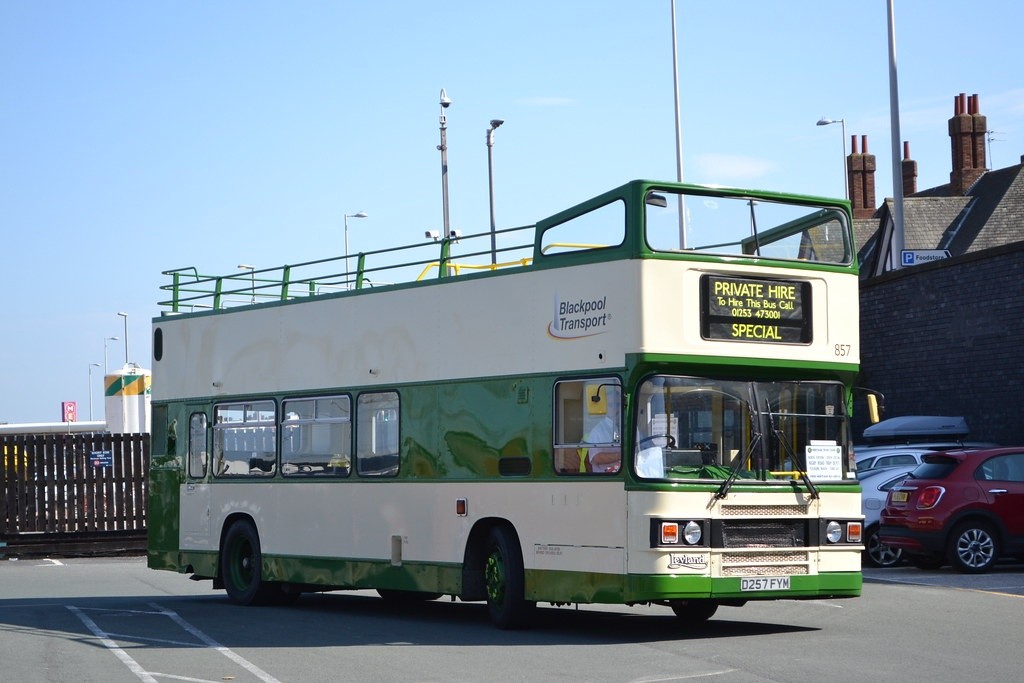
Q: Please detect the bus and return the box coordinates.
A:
[146,177,888,623]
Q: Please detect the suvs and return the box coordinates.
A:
[878,448,1024,573]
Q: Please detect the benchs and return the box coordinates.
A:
[223,451,395,476]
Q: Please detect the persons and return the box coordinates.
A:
[588,393,657,473]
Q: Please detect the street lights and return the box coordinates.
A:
[104,337,118,373]
[89,362,100,421]
[818,119,849,200]
[118,312,127,363]
[344,211,368,293]
[486,117,504,267]
[236,265,256,305]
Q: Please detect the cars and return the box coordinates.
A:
[29,466,105,525]
[779,414,1021,569]
